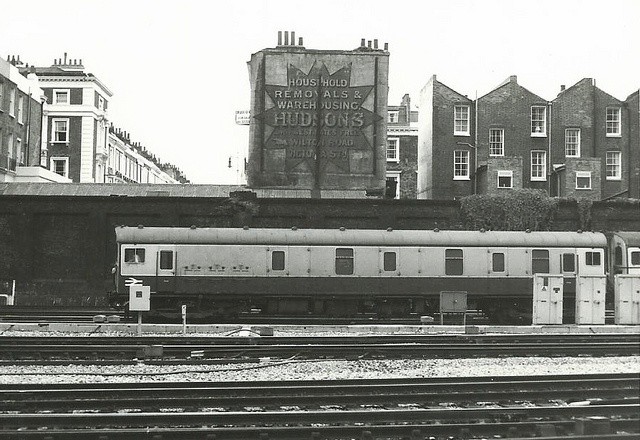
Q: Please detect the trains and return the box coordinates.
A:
[105,225,640,325]
